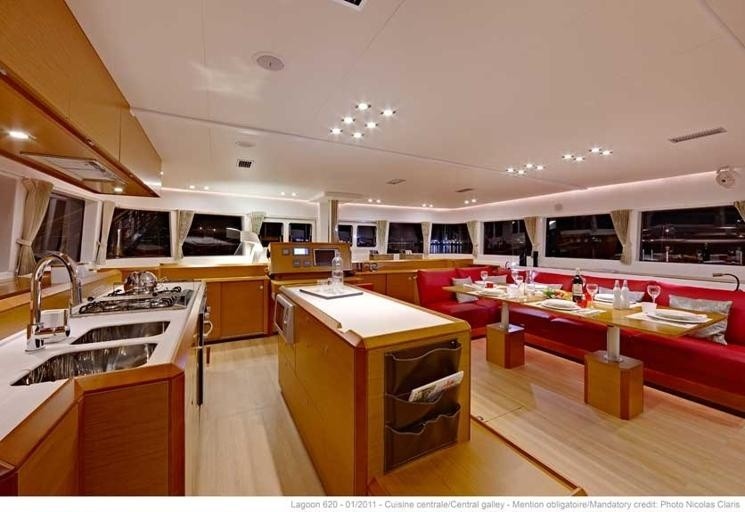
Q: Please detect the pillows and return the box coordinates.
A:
[452,275,733,345]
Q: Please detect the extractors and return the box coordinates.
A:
[20,152,128,194]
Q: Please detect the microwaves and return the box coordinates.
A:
[272,293,295,344]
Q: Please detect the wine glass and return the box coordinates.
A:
[473,260,537,300]
[647,285,662,303]
[585,283,599,309]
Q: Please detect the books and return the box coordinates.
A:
[407,369,464,404]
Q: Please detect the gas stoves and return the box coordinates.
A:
[69,285,194,318]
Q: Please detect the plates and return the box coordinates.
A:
[646,311,713,324]
[541,298,581,311]
[594,294,637,304]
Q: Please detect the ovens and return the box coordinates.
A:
[197,296,214,406]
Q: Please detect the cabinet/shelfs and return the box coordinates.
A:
[78,279,267,497]
[366,273,418,303]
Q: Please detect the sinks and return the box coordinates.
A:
[71,320,170,346]
[11,342,158,388]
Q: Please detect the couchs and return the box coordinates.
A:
[418,265,745,416]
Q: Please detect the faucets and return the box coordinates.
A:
[28,252,82,323]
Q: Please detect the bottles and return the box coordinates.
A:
[613,279,622,309]
[331,249,344,293]
[571,267,584,303]
[621,279,630,309]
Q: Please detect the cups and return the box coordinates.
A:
[641,302,657,314]
[315,277,335,294]
